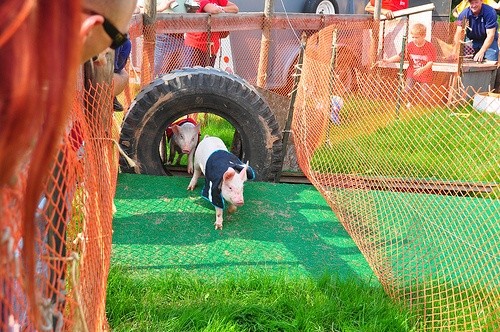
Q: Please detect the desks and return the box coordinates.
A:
[375,58,498,108]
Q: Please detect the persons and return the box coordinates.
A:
[454,0,499,63]
[0,0,239,332]
[365,0,409,20]
[381,23,436,108]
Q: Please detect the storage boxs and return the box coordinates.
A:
[473,92,500,113]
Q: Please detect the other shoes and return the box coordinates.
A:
[112,97,124,112]
[405,102,413,109]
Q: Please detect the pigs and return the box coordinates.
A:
[186,134,247,230]
[167,122,201,173]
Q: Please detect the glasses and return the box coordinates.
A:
[82,7,128,50]
